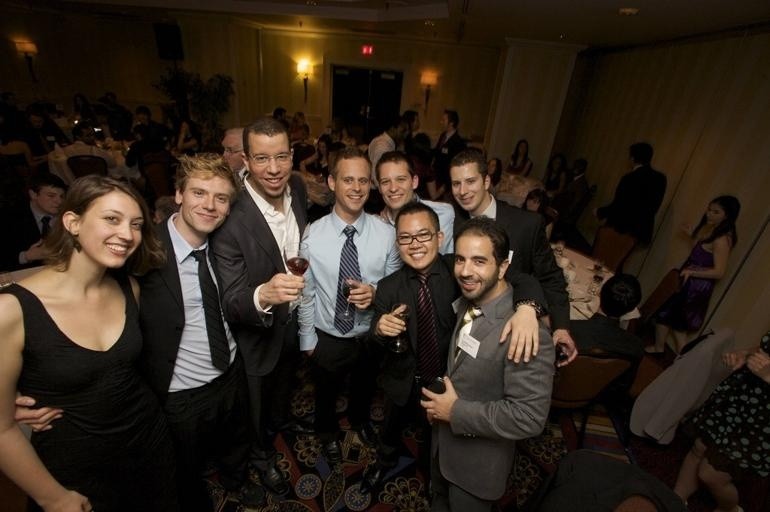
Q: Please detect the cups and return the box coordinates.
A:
[561,260,578,285]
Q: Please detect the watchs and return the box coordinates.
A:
[513,296,545,317]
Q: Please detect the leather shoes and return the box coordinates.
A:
[357,424,377,448]
[236,480,266,506]
[322,439,342,462]
[254,464,289,498]
[424,473,433,501]
[360,460,388,495]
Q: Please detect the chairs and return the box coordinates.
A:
[625,268,685,337]
[593,226,634,275]
[0,115,177,215]
[549,351,630,448]
[632,328,733,441]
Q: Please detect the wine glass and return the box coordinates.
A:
[589,258,606,294]
[282,241,316,307]
[335,277,361,323]
[388,304,413,356]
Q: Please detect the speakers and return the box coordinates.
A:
[155,22,184,61]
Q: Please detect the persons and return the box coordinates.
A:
[571,272,642,408]
[127,150,268,510]
[549,158,588,222]
[489,140,553,239]
[413,211,558,512]
[353,198,547,499]
[0,171,179,512]
[275,106,367,207]
[674,332,769,512]
[367,109,467,213]
[645,195,741,355]
[592,142,667,274]
[2,93,205,271]
[209,115,322,497]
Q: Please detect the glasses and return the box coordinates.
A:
[396,232,437,245]
[247,153,290,166]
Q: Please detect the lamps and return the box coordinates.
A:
[295,56,314,104]
[420,70,438,116]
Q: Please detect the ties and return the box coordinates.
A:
[455,302,482,359]
[334,225,361,335]
[41,216,51,239]
[415,273,441,384]
[188,246,229,370]
